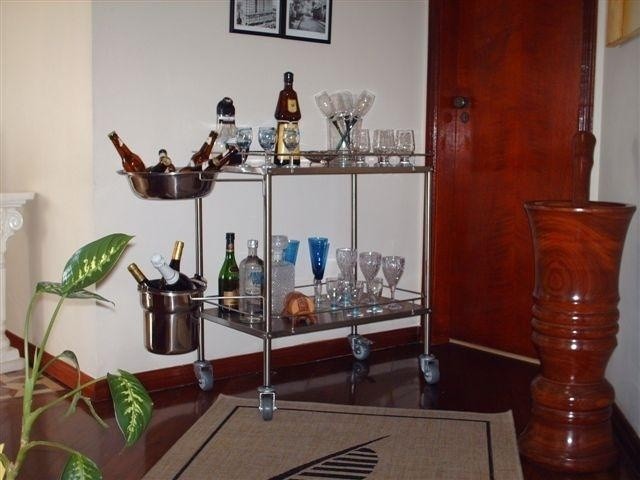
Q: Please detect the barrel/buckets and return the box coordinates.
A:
[138,278,206,356]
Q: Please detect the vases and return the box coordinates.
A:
[514,129,639,474]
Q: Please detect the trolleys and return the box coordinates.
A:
[117,152,440,421]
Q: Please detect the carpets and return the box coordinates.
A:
[138,393,526,480]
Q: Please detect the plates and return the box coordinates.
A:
[302,149,337,164]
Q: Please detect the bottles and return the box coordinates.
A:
[105,126,237,173]
[220,233,295,324]
[127,241,198,290]
[275,71,301,167]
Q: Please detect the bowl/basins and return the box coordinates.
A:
[116,168,222,200]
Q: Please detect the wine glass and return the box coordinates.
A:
[234,126,300,169]
[353,130,416,169]
[315,89,376,158]
[285,238,404,315]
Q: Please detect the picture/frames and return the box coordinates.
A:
[229,0,332,44]
